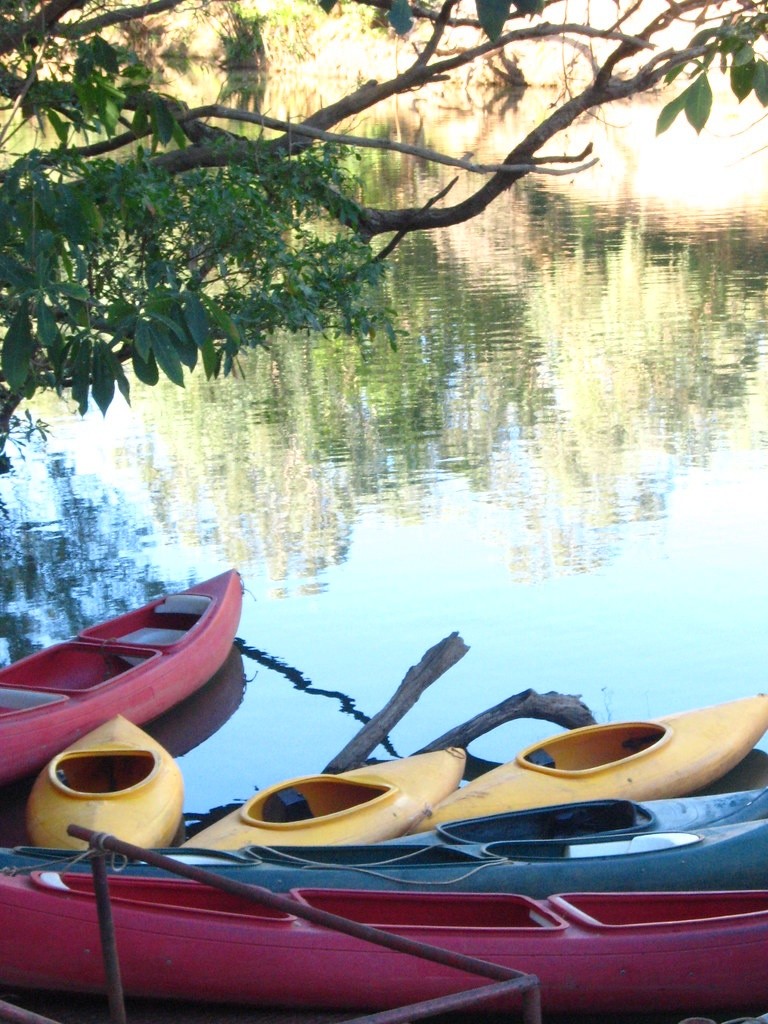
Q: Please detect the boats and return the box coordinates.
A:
[21,713,187,851]
[178,747,466,849]
[406,692,768,838]
[361,781,768,847]
[0,567,247,784]
[0,815,768,894]
[0,868,768,1014]
